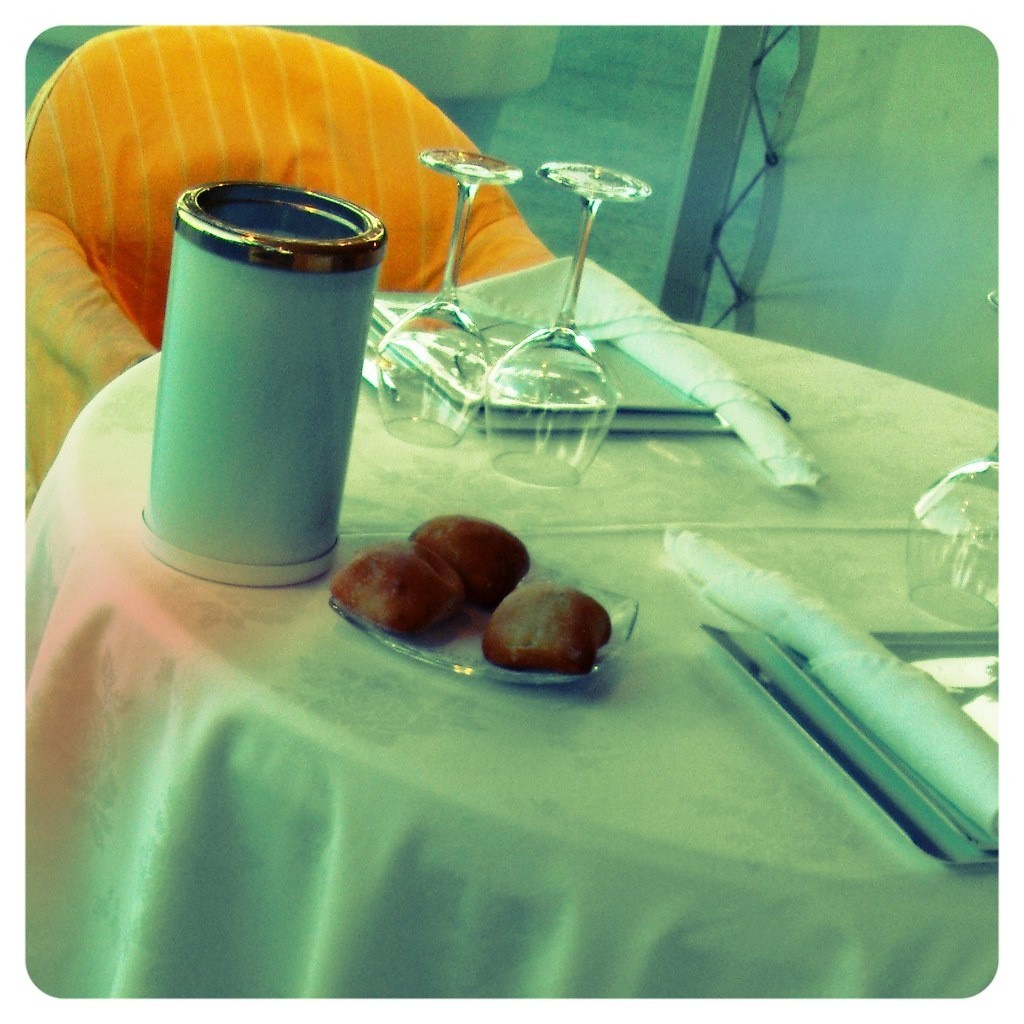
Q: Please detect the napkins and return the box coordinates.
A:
[464,259,828,487]
[664,523,999,840]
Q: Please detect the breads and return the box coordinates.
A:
[329,514,612,676]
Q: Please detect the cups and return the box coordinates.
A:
[139,178,387,589]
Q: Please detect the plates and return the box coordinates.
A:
[328,521,638,680]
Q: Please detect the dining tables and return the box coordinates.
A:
[29,294,1000,998]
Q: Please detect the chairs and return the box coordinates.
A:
[25,27,556,514]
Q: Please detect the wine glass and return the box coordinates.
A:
[375,149,523,448]
[487,163,653,488]
[907,285,998,628]
